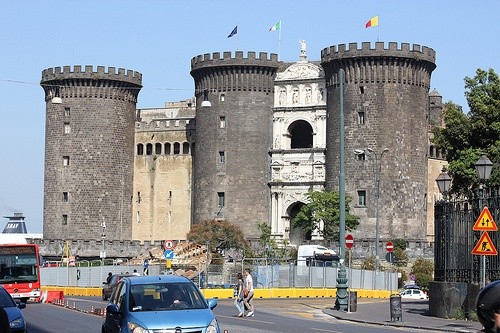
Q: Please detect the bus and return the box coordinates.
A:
[0,232,42,306]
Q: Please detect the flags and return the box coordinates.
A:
[228,26,237,38]
[269,21,280,32]
[366,16,378,28]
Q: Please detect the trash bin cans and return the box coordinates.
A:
[390,294,402,322]
[347,291,357,311]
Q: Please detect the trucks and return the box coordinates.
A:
[298,245,329,261]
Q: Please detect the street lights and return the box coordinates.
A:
[472,154,494,287]
[367,147,389,290]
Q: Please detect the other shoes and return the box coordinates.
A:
[246,311,253,316]
[251,314,254,317]
[238,312,243,316]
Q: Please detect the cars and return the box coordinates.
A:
[305,252,341,268]
[101,275,229,333]
[399,288,429,303]
[102,273,139,301]
[0,286,28,333]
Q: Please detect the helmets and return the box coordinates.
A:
[476,280,500,333]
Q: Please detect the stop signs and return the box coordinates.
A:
[345,234,353,248]
[385,241,393,252]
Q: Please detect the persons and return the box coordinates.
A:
[293,93,296,103]
[299,40,306,54]
[244,268,254,317]
[234,273,244,318]
[476,281,500,333]
[133,269,140,276]
[306,89,310,102]
[126,271,129,274]
[107,272,112,281]
[168,287,192,307]
[121,271,124,274]
[280,90,285,105]
[317,91,322,104]
